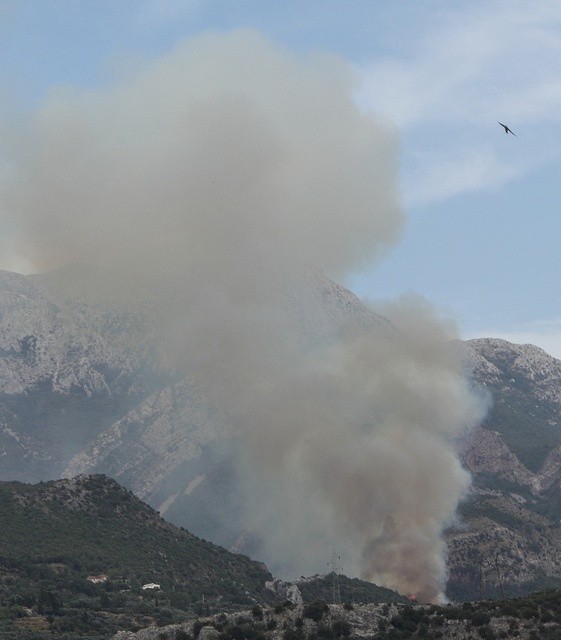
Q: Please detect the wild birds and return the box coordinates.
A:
[496,121,516,136]
